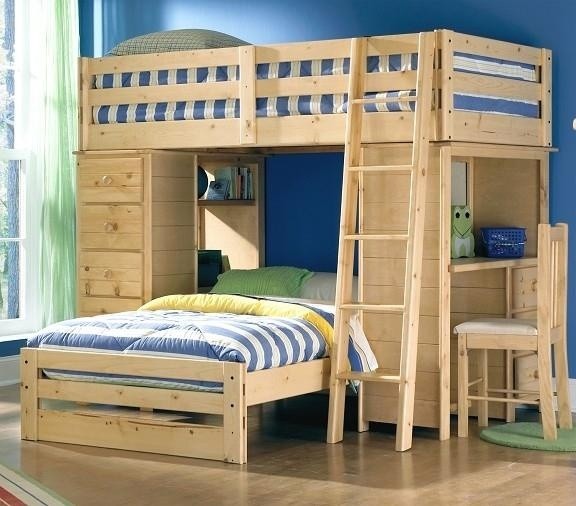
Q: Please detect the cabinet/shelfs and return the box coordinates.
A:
[71,149,266,319]
[358,142,559,440]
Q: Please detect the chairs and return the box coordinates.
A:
[451,223,572,440]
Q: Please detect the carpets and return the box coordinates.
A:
[0,457,77,506]
[480,421,576,453]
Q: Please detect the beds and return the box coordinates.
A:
[19,293,379,465]
[77,27,552,154]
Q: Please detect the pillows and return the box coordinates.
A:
[104,29,254,56]
[209,266,318,298]
[301,272,359,305]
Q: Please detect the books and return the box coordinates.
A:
[205,166,254,201]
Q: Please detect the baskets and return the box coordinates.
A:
[479,227,527,257]
